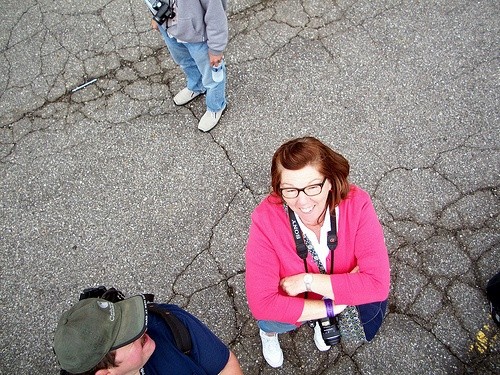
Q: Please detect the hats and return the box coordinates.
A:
[54,294,149,375]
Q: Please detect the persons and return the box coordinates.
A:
[146,0,228,132]
[246,136,390,368]
[54,295,245,375]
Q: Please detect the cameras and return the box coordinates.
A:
[307,315,342,346]
[152,0,176,26]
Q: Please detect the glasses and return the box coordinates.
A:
[279,176,327,199]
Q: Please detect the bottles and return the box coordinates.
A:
[211,57,223,82]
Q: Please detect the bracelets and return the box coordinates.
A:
[324,300,335,319]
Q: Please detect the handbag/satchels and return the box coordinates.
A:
[355,300,388,341]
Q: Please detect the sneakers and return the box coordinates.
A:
[259,328,285,368]
[313,321,332,351]
[198,104,228,132]
[173,87,205,106]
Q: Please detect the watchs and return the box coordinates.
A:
[303,271,315,292]
